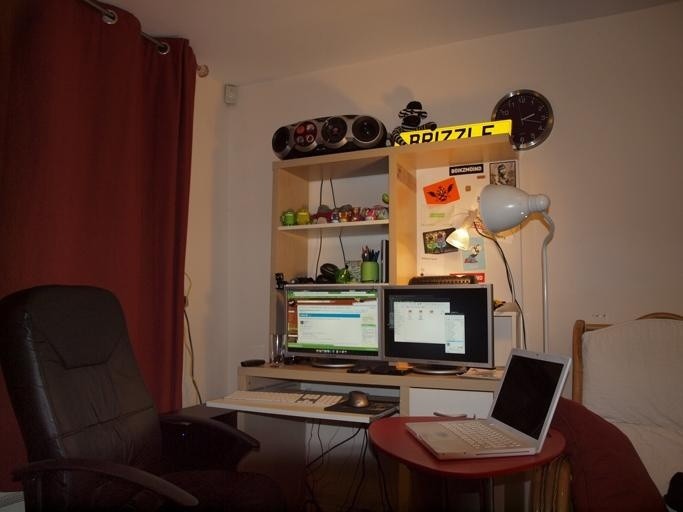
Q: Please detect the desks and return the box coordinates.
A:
[361,415,567,509]
[204,358,504,510]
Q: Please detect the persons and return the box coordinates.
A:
[434,232,446,252]
[497,163,510,186]
[425,234,436,251]
[468,243,481,262]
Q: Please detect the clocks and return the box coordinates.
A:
[489,86,554,150]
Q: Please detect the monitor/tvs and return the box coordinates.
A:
[284,284,381,368]
[381,283,496,374]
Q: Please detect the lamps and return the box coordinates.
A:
[445,208,530,353]
[478,183,557,354]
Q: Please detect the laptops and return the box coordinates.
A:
[405,348,572,460]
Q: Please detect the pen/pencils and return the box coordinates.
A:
[362,245,380,262]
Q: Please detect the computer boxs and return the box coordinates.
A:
[157,406,237,472]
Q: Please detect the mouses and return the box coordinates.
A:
[349,391,369,408]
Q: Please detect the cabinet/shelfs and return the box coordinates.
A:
[268,131,518,286]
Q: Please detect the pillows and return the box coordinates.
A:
[553,393,670,512]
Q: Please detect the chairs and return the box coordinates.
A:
[524,309,682,512]
[0,283,253,512]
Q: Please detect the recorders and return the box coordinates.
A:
[272,113,388,160]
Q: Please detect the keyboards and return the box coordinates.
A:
[223,390,343,407]
